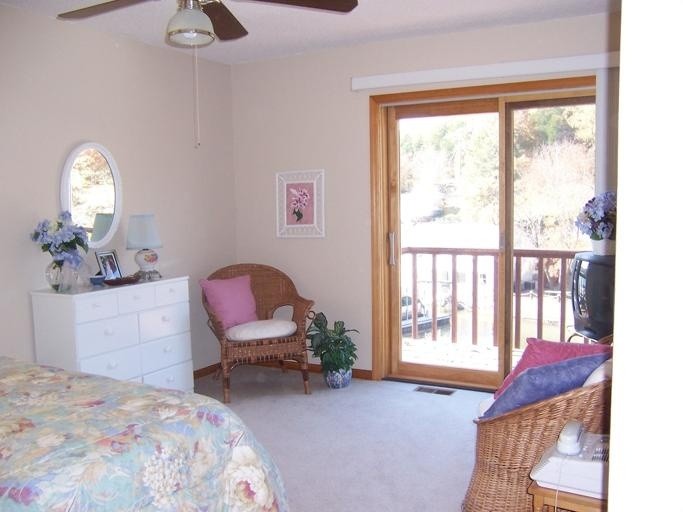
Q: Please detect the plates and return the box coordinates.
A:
[104,275,140,286]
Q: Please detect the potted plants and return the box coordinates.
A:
[303,310,361,392]
[44,258,80,292]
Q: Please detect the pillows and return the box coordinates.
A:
[200,274,260,332]
[478,349,612,421]
[583,356,613,388]
[492,335,613,400]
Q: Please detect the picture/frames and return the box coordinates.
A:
[274,168,326,239]
[94,248,125,280]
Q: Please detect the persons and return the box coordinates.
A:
[101,257,119,279]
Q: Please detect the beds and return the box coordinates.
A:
[1,354,292,511]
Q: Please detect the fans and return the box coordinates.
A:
[57,0,358,44]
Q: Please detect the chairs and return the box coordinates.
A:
[459,331,613,511]
[199,261,317,405]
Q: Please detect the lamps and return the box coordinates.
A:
[125,210,165,282]
[89,210,115,242]
[165,0,217,51]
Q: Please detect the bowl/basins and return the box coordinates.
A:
[89,275,107,285]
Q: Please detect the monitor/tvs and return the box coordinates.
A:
[571,253,616,340]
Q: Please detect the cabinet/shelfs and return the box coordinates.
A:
[28,273,197,394]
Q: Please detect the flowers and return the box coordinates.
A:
[573,190,617,239]
[28,209,91,271]
[288,187,310,222]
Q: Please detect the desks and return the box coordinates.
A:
[525,479,609,511]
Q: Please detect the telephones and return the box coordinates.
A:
[530,419,610,501]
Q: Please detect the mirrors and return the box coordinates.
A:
[59,142,124,251]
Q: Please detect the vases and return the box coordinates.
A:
[591,238,616,257]
